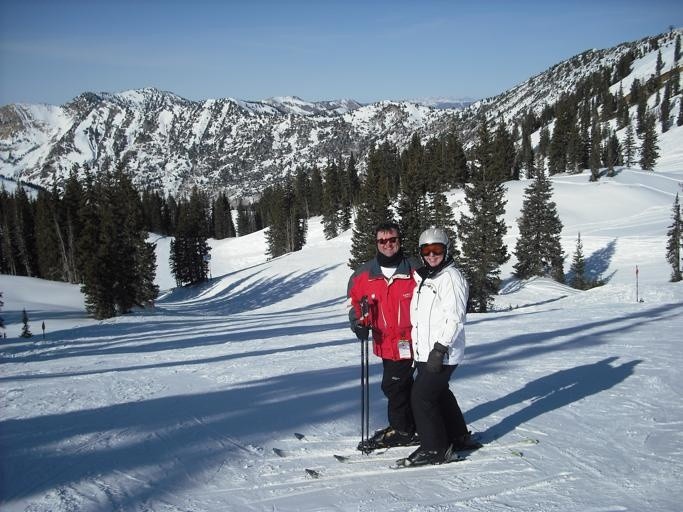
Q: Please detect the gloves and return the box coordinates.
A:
[355,325,368,340]
[426,341,449,374]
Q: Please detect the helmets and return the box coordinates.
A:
[418,228,450,266]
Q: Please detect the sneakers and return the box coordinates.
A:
[408,431,473,465]
[374,427,420,445]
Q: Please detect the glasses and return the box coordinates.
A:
[421,243,445,256]
[376,237,399,244]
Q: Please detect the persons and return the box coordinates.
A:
[399,228,472,467]
[347,222,423,446]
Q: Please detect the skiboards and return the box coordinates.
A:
[272,431,500,457]
[304,439,539,479]
